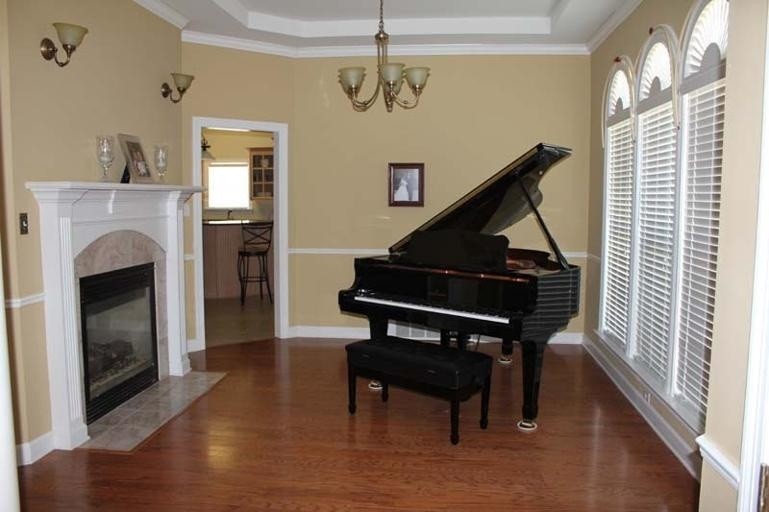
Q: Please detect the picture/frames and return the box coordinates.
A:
[388,163,424,207]
[117,133,155,184]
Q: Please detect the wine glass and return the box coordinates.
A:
[95,135,115,183]
[155,145,169,185]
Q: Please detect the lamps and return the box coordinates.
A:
[160,72,195,104]
[200,130,215,161]
[337,0,430,112]
[41,22,89,67]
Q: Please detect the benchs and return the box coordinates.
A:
[345,335,493,444]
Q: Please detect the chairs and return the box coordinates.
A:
[237,220,273,306]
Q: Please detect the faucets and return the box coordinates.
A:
[227,210,233,219]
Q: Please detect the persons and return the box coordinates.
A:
[394,173,413,201]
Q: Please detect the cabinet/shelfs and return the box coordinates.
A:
[246,147,273,201]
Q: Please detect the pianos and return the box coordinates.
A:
[338,142,579,421]
